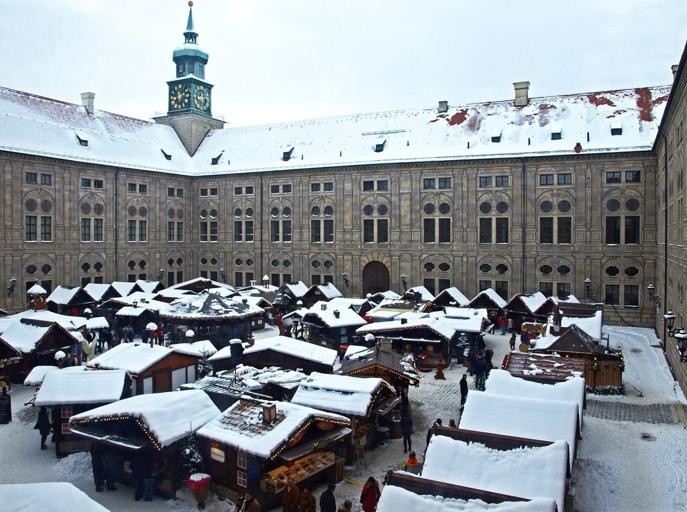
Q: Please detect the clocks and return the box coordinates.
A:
[170,84,190,110]
[193,85,210,111]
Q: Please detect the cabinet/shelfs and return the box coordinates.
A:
[259,452,335,511]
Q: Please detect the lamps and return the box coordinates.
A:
[8,276,17,297]
[664,308,676,337]
[585,277,591,298]
[400,273,407,290]
[219,268,227,283]
[675,328,687,363]
[158,267,165,281]
[647,283,655,296]
[342,272,349,288]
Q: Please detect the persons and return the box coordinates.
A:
[471,342,493,391]
[90,439,168,501]
[400,396,414,452]
[433,418,457,427]
[510,330,530,351]
[38,406,66,458]
[235,477,381,512]
[54,345,82,368]
[70,305,164,350]
[460,374,468,407]
[404,452,422,474]
[490,313,507,335]
